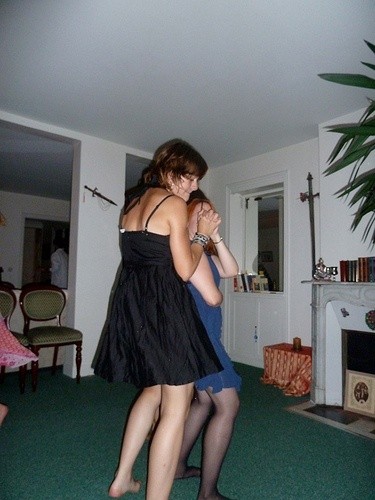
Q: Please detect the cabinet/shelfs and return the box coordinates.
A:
[227,291,288,369]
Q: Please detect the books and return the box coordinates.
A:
[234,272,257,293]
[356,256,375,282]
[340,260,356,282]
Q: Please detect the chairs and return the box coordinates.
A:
[0,285,29,395]
[20,282,82,392]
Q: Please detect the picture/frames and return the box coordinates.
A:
[261,251,273,262]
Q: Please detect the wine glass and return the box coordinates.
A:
[324,267,338,282]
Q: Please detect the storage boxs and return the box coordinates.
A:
[263,343,312,395]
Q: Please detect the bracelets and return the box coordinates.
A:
[212,236,223,244]
[192,232,209,248]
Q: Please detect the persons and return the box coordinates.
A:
[49,239,67,290]
[173,197,242,500]
[0,308,39,425]
[90,137,225,500]
